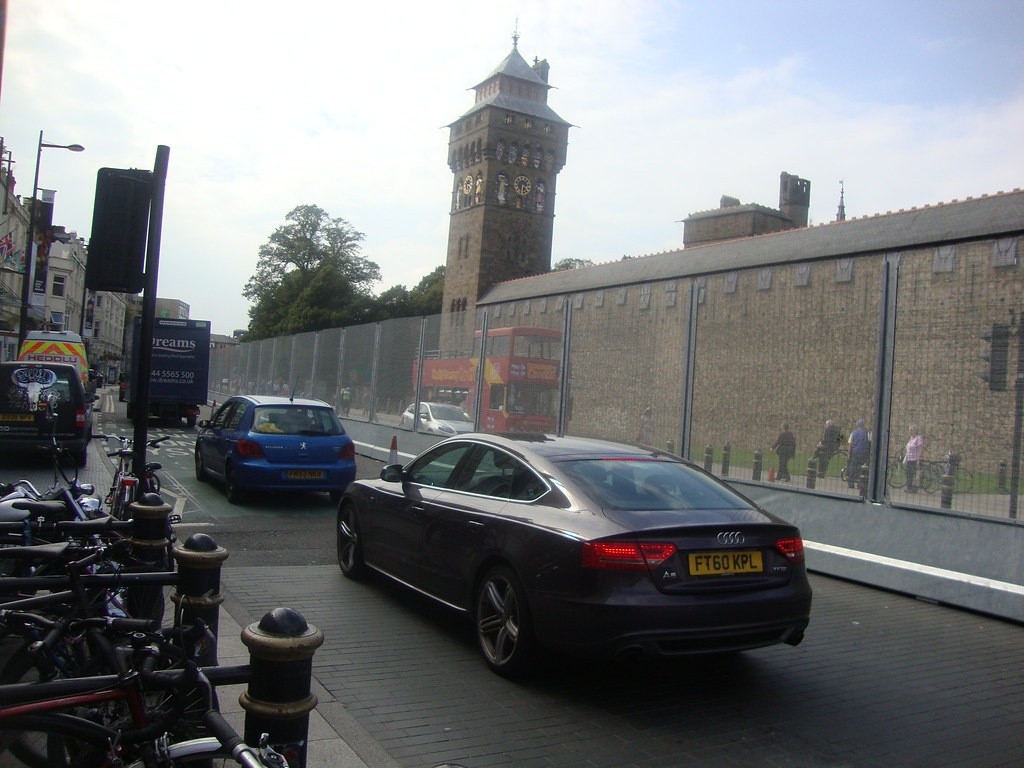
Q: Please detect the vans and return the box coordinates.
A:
[17,329,95,394]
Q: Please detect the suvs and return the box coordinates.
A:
[0,358,96,467]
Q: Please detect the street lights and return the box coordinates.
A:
[0,128,86,361]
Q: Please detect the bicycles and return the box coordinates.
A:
[886,446,974,495]
[0,399,291,768]
[811,440,870,481]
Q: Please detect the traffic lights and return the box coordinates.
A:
[976,320,1010,393]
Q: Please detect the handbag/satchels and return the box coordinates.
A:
[911,462,923,487]
[902,454,908,464]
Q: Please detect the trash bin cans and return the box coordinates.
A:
[96,375,104,388]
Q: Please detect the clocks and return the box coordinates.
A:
[514,175,531,196]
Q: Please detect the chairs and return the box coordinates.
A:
[636,475,692,507]
[574,461,615,496]
[468,450,519,496]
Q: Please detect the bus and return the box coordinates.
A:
[411,325,576,437]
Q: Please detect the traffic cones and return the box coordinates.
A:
[766,466,775,483]
[210,398,218,419]
[386,435,399,465]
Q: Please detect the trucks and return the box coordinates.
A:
[116,315,212,428]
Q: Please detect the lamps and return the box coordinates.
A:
[463,175,473,194]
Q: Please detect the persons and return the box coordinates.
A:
[904,426,925,493]
[638,406,658,446]
[340,386,351,417]
[847,418,871,489]
[770,422,795,483]
[816,419,841,478]
[247,379,288,397]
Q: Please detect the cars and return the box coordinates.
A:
[193,394,358,506]
[334,429,814,685]
[398,400,473,438]
